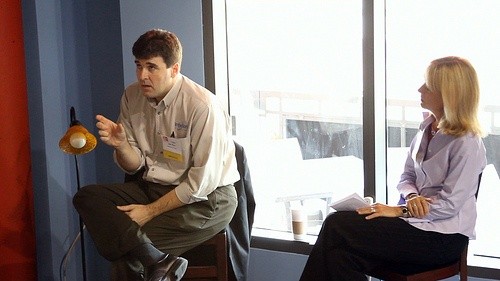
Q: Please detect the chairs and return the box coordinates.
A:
[180,140,246,281]
[366,172,483,281]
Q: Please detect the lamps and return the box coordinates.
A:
[59,107,98,281]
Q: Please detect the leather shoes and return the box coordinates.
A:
[144,253,188,281]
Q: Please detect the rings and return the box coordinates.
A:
[412,202,416,206]
[371,208,376,213]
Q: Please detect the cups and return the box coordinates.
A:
[292,208,305,240]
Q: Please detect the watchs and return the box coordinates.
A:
[402,206,408,218]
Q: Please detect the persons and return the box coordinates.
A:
[299,56,487,281]
[73,29,240,281]
